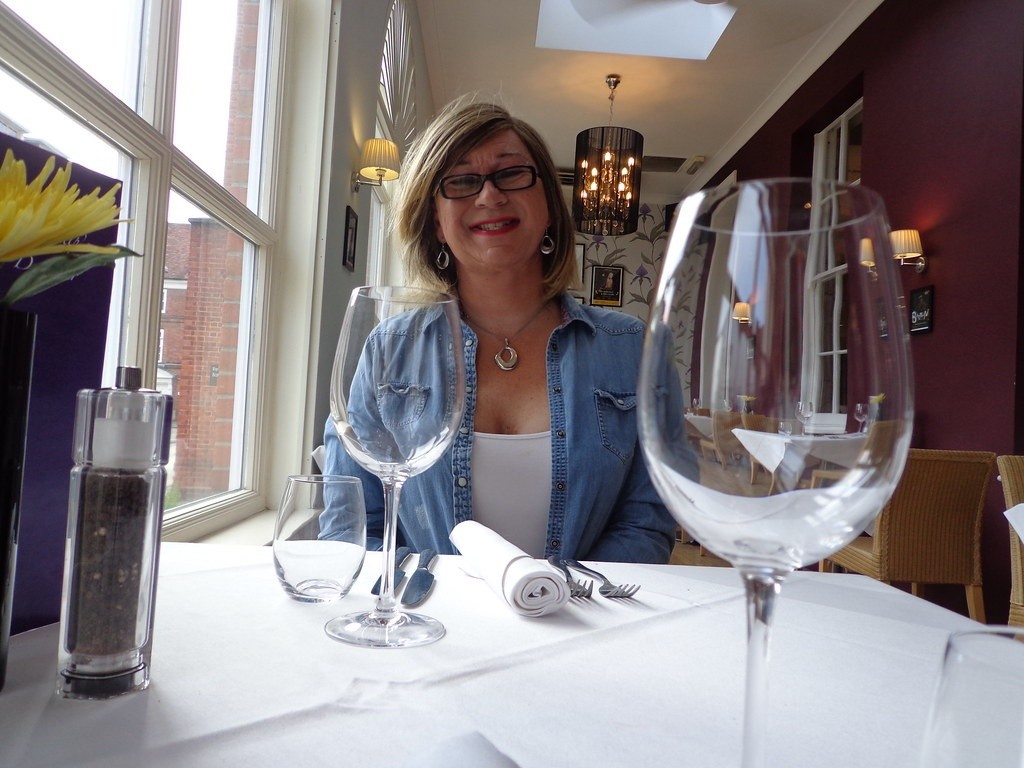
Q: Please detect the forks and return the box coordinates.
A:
[564,557,641,598]
[547,554,593,597]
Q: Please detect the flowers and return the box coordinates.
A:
[0,147,145,305]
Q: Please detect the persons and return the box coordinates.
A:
[318,102,700,565]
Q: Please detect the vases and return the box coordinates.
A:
[1,312,37,691]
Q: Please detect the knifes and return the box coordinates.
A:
[402,549,438,605]
[372,544,410,596]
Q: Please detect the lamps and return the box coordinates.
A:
[351,138,399,193]
[572,74,645,236]
[731,302,751,323]
[888,230,928,274]
[859,237,879,282]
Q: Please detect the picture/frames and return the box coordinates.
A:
[908,285,934,334]
[568,243,585,290]
[575,296,585,304]
[590,264,624,308]
[342,205,358,273]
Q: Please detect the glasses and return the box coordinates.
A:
[430,164,543,200]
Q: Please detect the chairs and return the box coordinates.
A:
[997,456,1024,640]
[819,448,996,626]
[683,406,711,417]
[811,419,904,489]
[699,412,745,470]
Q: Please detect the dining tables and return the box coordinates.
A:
[0,541,1024,768]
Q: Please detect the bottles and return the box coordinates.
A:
[58,364,173,698]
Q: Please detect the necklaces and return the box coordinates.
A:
[455,298,557,371]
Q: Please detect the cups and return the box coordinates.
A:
[272,476,366,603]
[920,627,1024,768]
[777,421,792,436]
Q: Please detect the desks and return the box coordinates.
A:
[741,414,779,485]
[684,414,713,442]
[731,428,868,493]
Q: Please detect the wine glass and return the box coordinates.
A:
[853,403,868,436]
[693,398,702,416]
[325,283,466,647]
[795,401,815,437]
[635,178,913,766]
[722,400,733,412]
